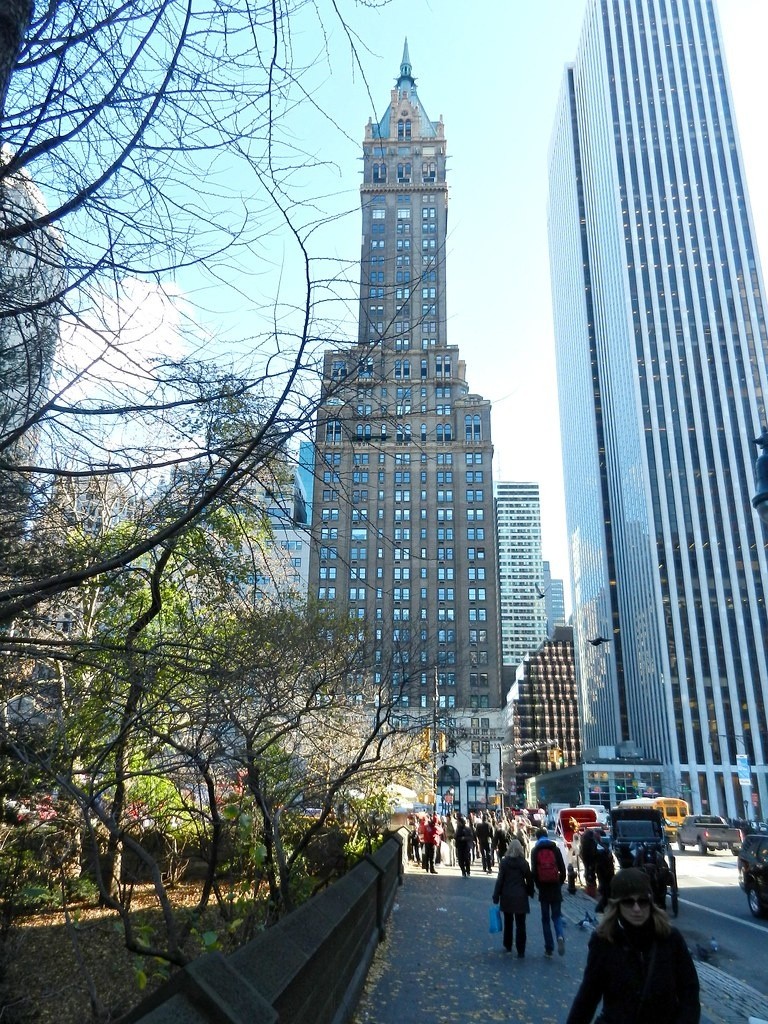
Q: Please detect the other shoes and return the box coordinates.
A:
[545,951,553,958]
[517,952,526,959]
[504,946,513,952]
[557,936,566,957]
[431,870,438,874]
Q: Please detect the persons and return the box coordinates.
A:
[409,807,752,962]
[566,868,703,1024]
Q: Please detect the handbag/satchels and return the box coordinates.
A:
[488,896,502,933]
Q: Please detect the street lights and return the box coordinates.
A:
[743,800,753,821]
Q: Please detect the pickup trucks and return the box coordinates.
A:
[677,815,743,856]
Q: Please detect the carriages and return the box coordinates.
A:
[546,803,679,917]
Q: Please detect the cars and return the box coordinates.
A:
[738,834,768,917]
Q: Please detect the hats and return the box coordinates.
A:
[611,868,652,899]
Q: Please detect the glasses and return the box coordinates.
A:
[618,893,653,909]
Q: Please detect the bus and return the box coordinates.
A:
[612,797,693,837]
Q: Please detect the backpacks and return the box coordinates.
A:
[537,845,559,882]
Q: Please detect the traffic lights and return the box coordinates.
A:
[550,747,562,762]
